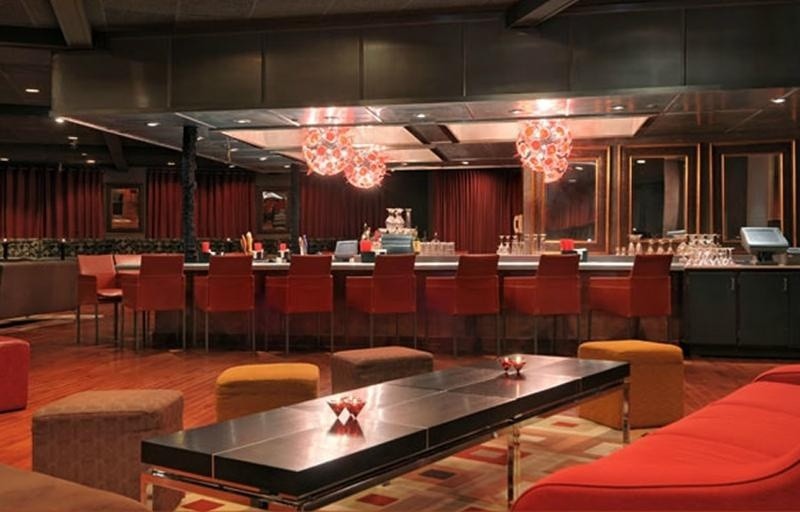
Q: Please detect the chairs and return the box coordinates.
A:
[426,254,501,358]
[114,255,143,347]
[118,256,186,352]
[587,255,671,344]
[346,254,417,349]
[265,255,334,354]
[76,255,123,346]
[504,254,581,355]
[193,255,256,351]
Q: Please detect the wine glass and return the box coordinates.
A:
[679,234,736,268]
[385,208,405,229]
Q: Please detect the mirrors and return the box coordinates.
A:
[255,186,294,234]
[103,183,146,233]
[617,144,700,254]
[530,146,610,255]
[709,140,797,254]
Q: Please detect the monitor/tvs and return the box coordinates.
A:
[333,240,359,258]
[740,225,790,264]
[380,235,413,253]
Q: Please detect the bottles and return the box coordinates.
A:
[496,233,546,256]
[616,234,674,256]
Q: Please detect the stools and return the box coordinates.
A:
[32,390,184,502]
[1,337,28,413]
[330,346,433,395]
[216,363,319,423]
[577,340,683,428]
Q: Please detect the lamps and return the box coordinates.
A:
[302,128,353,176]
[344,146,386,188]
[515,119,571,171]
[542,158,568,183]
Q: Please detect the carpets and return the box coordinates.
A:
[146,414,638,511]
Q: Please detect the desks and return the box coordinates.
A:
[142,355,631,511]
[683,266,800,359]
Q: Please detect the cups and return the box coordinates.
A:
[210,249,289,263]
[420,242,455,256]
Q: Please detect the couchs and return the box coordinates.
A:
[512,366,799,511]
[0,260,80,323]
[1,464,149,510]
